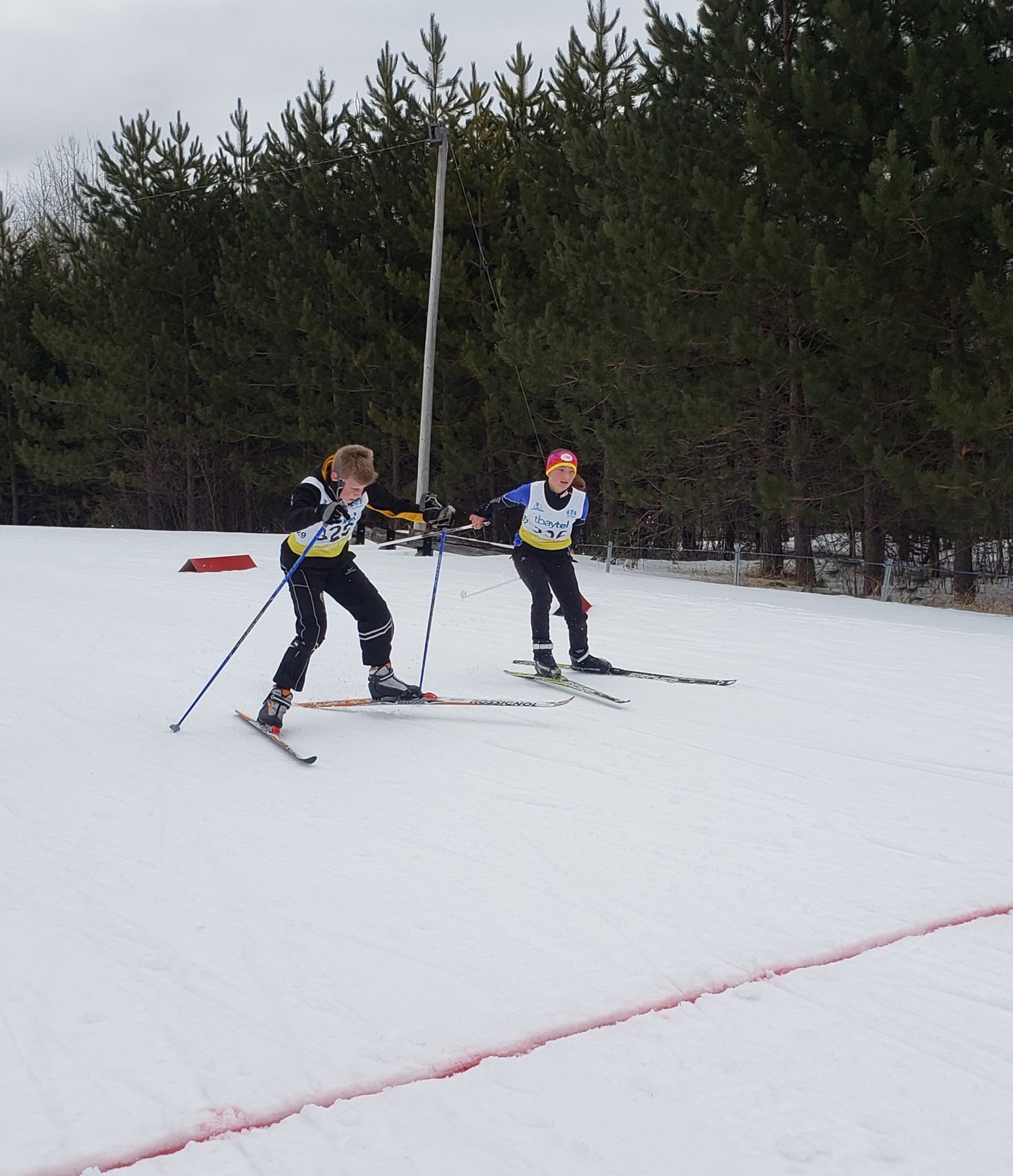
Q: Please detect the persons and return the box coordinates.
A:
[469,448,612,682]
[256,445,456,735]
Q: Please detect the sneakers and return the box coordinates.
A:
[368,665,420,702]
[258,689,293,728]
[532,640,561,677]
[568,648,611,673]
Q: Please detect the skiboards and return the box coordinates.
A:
[504,660,738,704]
[235,692,576,763]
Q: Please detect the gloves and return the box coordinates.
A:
[316,502,350,524]
[422,493,451,528]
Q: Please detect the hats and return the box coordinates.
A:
[545,451,577,476]
